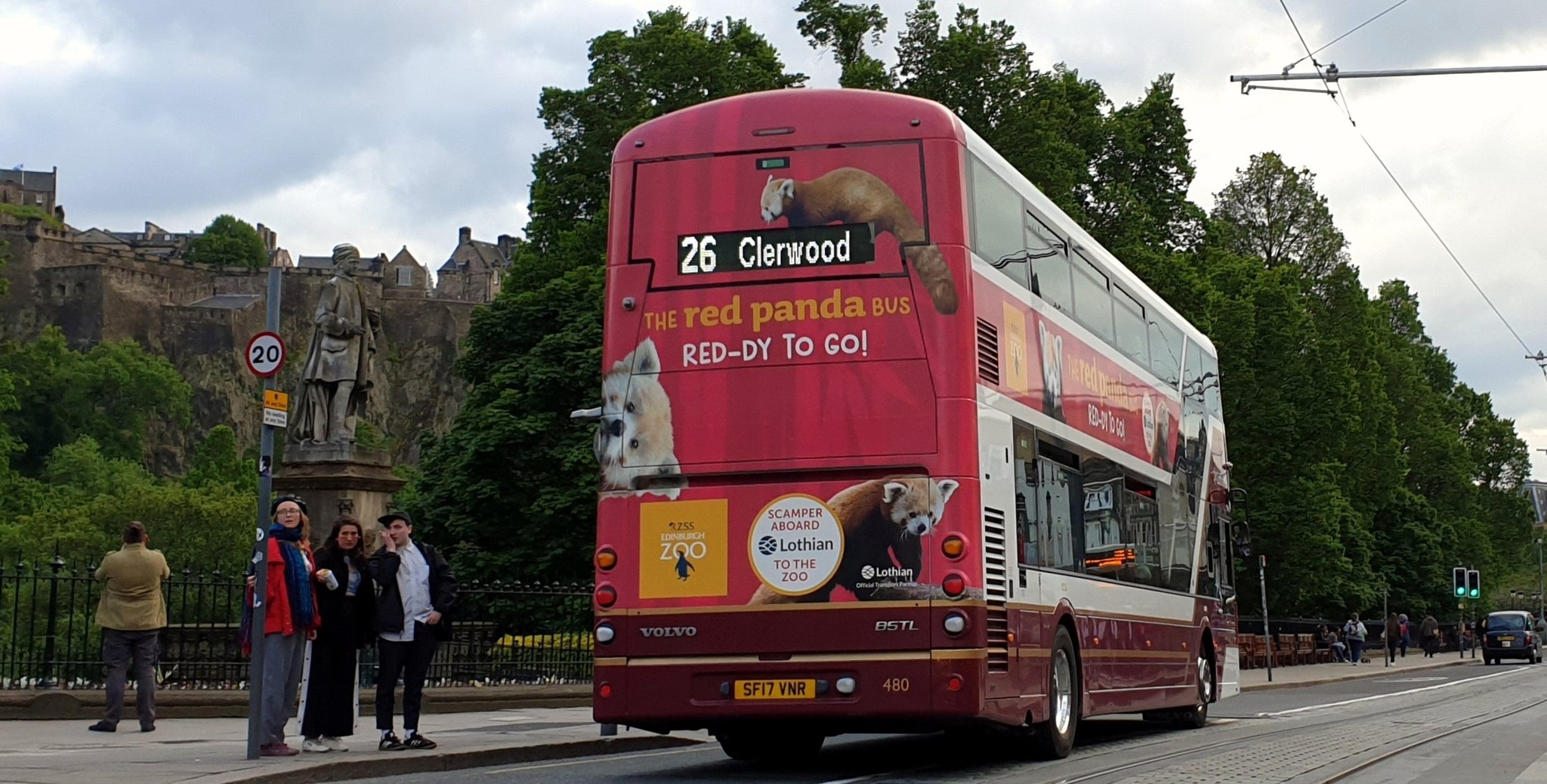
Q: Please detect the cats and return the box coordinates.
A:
[748,471,957,598]
[760,167,961,315]
[601,336,684,501]
[1152,402,1175,474]
[1038,319,1068,425]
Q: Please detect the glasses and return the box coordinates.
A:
[277,509,300,514]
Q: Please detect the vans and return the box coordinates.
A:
[1479,610,1544,666]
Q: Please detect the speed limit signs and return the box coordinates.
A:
[245,331,287,378]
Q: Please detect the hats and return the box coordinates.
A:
[377,511,411,525]
[271,495,308,516]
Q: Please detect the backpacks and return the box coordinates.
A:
[1401,621,1408,636]
[1349,621,1359,635]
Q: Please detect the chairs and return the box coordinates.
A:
[1237,632,1333,669]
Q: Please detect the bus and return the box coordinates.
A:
[591,85,1253,773]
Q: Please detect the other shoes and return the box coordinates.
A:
[1353,662,1358,666]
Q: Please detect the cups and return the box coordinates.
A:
[322,569,339,591]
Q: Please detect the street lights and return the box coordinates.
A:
[1510,590,1517,611]
[1531,593,1537,616]
[1518,591,1524,610]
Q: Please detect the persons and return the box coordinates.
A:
[1419,611,1438,658]
[1315,623,1351,663]
[369,508,457,750]
[1343,613,1367,665]
[246,495,320,755]
[88,522,170,733]
[1475,617,1483,649]
[1449,625,1472,651]
[314,243,377,440]
[300,516,371,753]
[1387,612,1411,666]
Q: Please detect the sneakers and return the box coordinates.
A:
[379,736,403,750]
[141,722,156,732]
[89,720,117,732]
[302,739,329,752]
[320,736,349,751]
[260,741,300,755]
[403,731,437,749]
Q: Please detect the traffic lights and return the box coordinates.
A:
[1467,570,1480,599]
[1453,567,1466,598]
